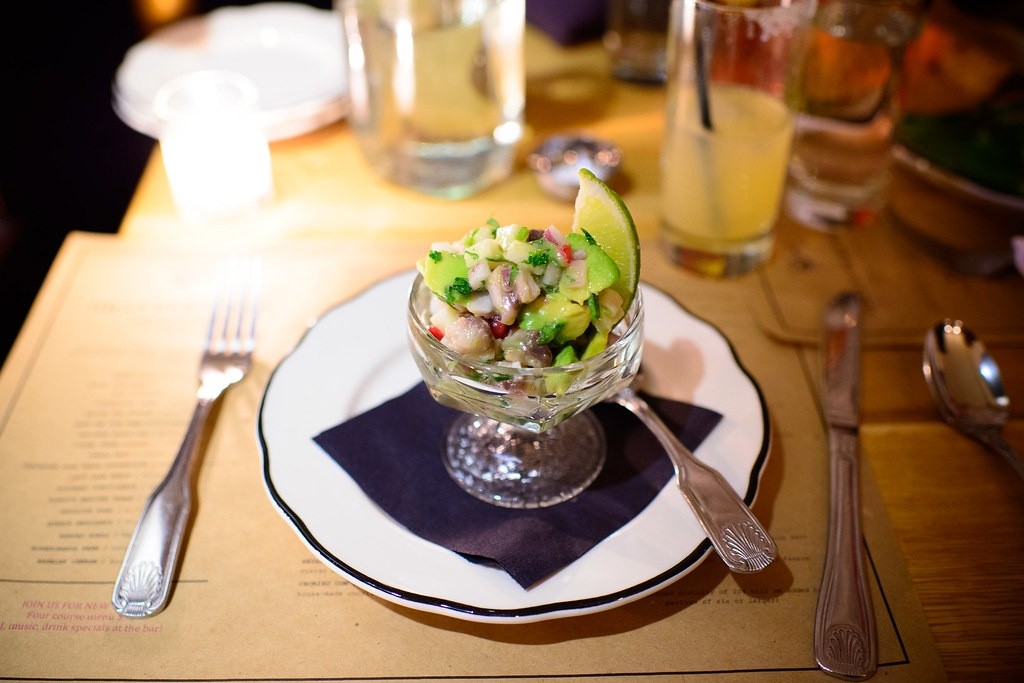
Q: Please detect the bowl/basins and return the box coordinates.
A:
[889,143,1024,259]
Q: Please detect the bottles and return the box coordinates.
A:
[784,0,900,232]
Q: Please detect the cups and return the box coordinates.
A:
[346,0,526,195]
[658,0,803,283]
[604,0,672,85]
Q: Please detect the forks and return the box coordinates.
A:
[111,295,258,619]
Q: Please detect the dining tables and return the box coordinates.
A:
[122,22,1024,683]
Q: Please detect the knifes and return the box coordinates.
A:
[814,290,877,677]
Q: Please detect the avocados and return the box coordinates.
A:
[423,218,620,396]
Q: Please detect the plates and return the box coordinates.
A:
[114,4,350,144]
[259,263,773,623]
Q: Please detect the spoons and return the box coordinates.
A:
[921,316,1024,488]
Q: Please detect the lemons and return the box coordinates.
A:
[572,169,641,330]
